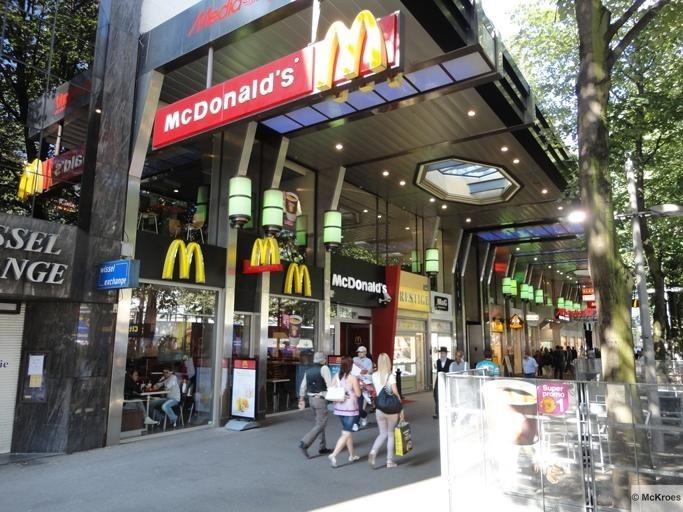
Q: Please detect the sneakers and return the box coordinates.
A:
[387,461,397,468]
[368,452,375,465]
[361,417,367,426]
[299,440,310,457]
[349,455,360,462]
[319,448,331,454]
[352,423,359,431]
[328,454,338,468]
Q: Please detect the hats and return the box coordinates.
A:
[438,346,449,352]
[357,346,367,352]
[313,351,326,363]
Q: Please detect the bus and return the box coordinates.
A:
[268,325,312,349]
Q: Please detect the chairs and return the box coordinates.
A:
[163,392,186,431]
[528,396,653,475]
[137,199,206,246]
[123,398,149,436]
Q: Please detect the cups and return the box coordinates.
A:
[140,383,151,391]
[481,380,539,450]
[284,191,298,223]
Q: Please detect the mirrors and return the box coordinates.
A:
[1,1,109,226]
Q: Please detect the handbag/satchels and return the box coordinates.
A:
[394,422,413,456]
[375,386,403,413]
[325,387,345,402]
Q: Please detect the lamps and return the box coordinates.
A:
[260,186,285,236]
[321,209,343,251]
[411,248,422,273]
[195,186,209,222]
[424,247,441,276]
[294,215,309,247]
[228,173,251,229]
[501,275,581,311]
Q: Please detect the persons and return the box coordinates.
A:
[298,350,333,461]
[129,344,644,432]
[329,352,361,469]
[367,352,405,469]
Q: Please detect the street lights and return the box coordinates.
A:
[614,151,683,455]
[644,284,682,383]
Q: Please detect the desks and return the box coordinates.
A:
[266,378,292,413]
[139,391,172,431]
